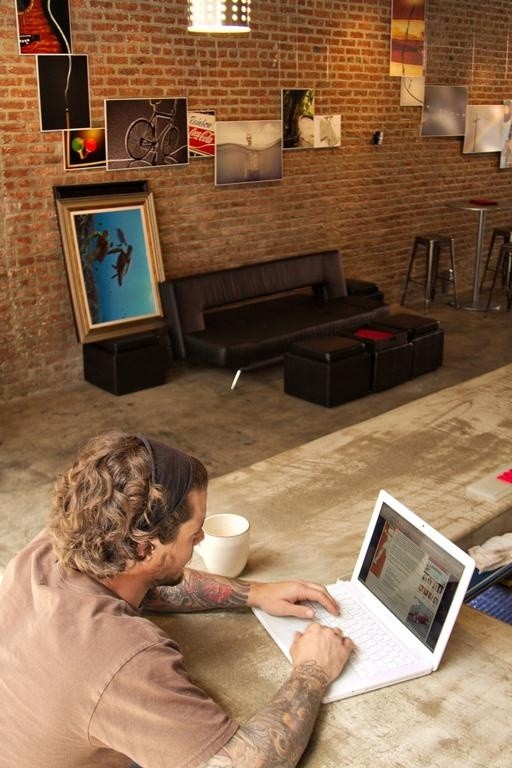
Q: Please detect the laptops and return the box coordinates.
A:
[251,488,477,706]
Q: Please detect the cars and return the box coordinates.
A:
[408,611,429,625]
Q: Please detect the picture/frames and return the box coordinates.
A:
[56,192,170,345]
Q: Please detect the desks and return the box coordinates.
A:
[444,199,502,312]
[145,362,512,768]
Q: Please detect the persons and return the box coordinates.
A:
[373,525,396,565]
[0,429,353,768]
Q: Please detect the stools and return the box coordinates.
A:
[479,226,512,295]
[283,336,373,408]
[84,330,169,396]
[486,243,511,312]
[400,232,461,314]
[339,323,414,393]
[376,313,444,377]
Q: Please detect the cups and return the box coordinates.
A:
[194,512,252,579]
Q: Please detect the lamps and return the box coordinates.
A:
[185,0,253,34]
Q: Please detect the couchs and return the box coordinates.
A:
[159,249,392,391]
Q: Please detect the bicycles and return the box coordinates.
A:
[126,155,178,168]
[124,98,180,162]
